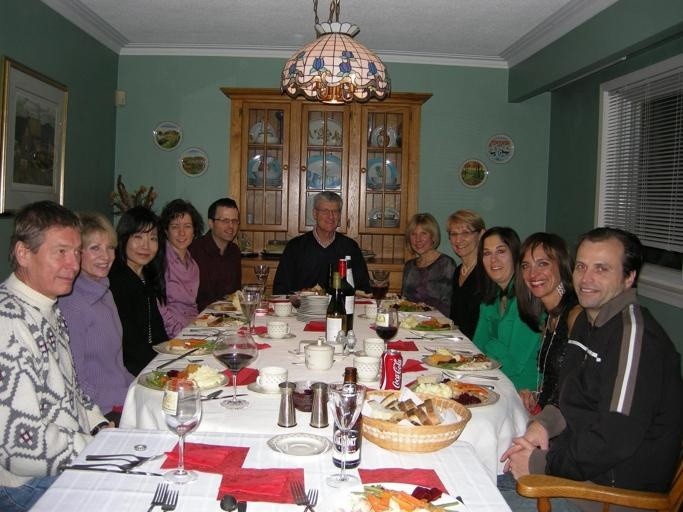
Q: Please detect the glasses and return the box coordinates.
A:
[449,229,482,237]
[214,217,241,226]
[316,208,342,215]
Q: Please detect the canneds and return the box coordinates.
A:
[380,350,402,390]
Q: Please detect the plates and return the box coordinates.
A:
[247,115,401,229]
[138,254,506,457]
[153,120,182,153]
[339,481,465,510]
[485,133,516,166]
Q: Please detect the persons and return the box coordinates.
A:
[155,198,201,340]
[106,204,171,374]
[446,210,486,340]
[272,191,371,295]
[187,198,241,314]
[400,213,458,318]
[55,210,138,428]
[499,226,683,512]
[515,231,586,416]
[0,200,115,511]
[473,226,549,394]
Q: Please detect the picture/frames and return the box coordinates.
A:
[487,135,514,164]
[152,122,181,152]
[0,58,69,216]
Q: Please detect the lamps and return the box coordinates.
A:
[280,0,391,105]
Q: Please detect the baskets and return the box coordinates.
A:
[361,389,472,454]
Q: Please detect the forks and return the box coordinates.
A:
[59,449,165,479]
[304,488,319,512]
[161,490,179,512]
[288,479,315,512]
[147,481,169,511]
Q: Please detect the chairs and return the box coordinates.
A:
[517,462,683,512]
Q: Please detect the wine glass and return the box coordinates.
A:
[327,383,364,493]
[159,377,204,483]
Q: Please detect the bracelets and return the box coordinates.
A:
[90,422,109,436]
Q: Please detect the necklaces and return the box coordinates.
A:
[461,259,477,276]
[535,313,560,404]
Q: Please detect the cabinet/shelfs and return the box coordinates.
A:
[220,85,433,291]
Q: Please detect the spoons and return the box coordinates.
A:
[220,495,238,512]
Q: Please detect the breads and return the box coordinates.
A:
[367,394,442,426]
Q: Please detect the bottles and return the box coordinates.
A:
[333,364,363,468]
